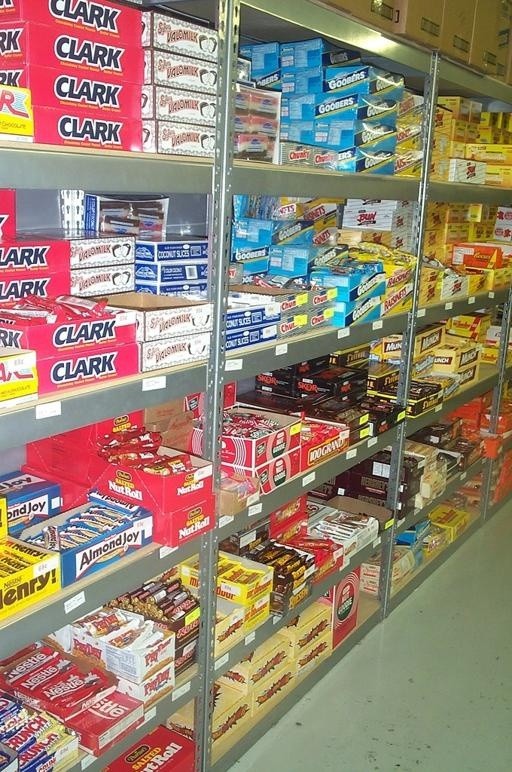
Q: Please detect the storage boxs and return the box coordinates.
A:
[317,0,511,82]
